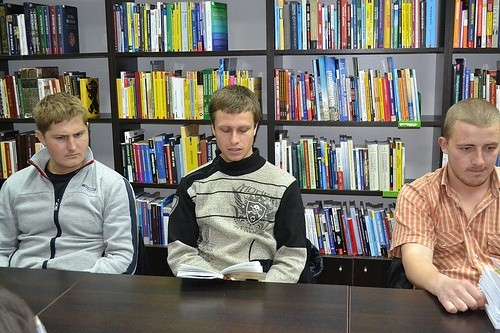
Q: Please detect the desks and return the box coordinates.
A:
[0,267,89,315]
[38,274,348,333]
[348,287,500,333]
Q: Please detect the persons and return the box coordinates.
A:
[167,85,307,283]
[389,98,500,313]
[0,92,138,275]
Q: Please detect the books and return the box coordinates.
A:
[0,2,80,56]
[275,0,439,50]
[116,58,262,120]
[136,192,175,247]
[453,0,500,48]
[275,130,405,191]
[0,130,44,179]
[176,261,266,279]
[113,1,228,52]
[121,124,217,184]
[304,200,396,257]
[452,58,500,110]
[0,66,100,118]
[274,55,420,121]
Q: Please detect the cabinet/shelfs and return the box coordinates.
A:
[0,0,121,192]
[268,0,446,257]
[107,0,267,245]
[446,0,500,166]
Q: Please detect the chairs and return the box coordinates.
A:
[299,256,352,285]
[352,259,415,289]
[136,245,175,277]
